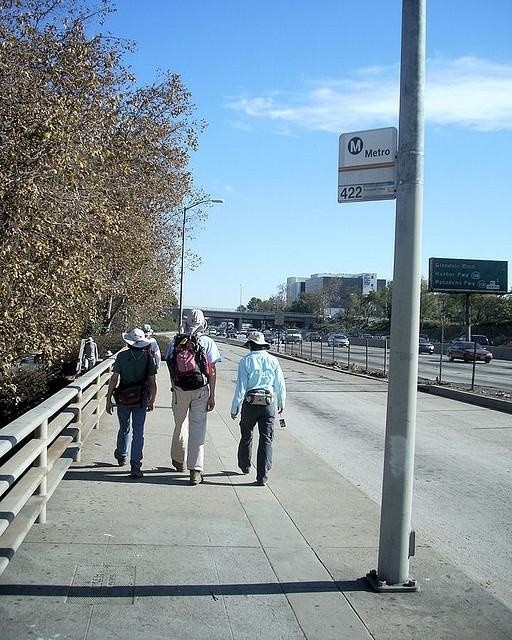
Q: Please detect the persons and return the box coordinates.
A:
[106,328,158,478]
[165,309,222,485]
[230,331,287,486]
[103,350,113,361]
[82,336,98,372]
[141,324,161,370]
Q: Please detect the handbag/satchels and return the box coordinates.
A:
[245,388,275,406]
[113,381,147,405]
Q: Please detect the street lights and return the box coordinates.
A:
[178,199,224,331]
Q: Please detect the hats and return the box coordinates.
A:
[122,328,151,348]
[182,309,207,333]
[240,331,271,350]
[87,337,95,342]
[141,324,154,336]
[105,350,113,356]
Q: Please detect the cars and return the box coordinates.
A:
[205,322,337,344]
[345,332,390,340]
[448,341,493,364]
[327,334,350,348]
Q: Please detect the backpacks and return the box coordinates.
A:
[167,333,210,391]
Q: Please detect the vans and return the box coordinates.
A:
[419,335,429,342]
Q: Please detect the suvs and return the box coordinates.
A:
[451,335,489,344]
[419,337,434,354]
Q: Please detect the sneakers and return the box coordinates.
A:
[172,459,187,472]
[242,468,250,473]
[114,448,129,466]
[190,470,204,486]
[256,477,269,486]
[131,467,143,479]
